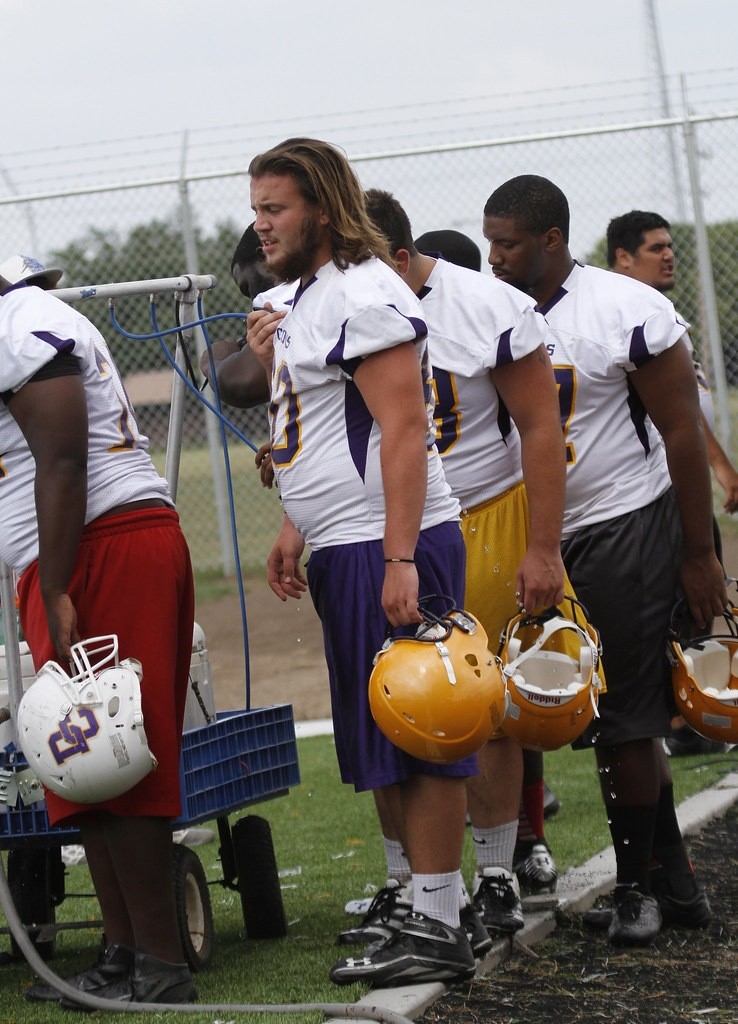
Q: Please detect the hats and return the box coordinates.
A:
[0,254,63,296]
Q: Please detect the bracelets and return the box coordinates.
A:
[385,558,415,563]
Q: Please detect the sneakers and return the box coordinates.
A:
[330,910,477,988]
[463,783,561,827]
[59,950,200,1012]
[470,866,524,935]
[343,896,376,916]
[516,838,557,894]
[581,893,616,929]
[336,876,415,945]
[650,886,713,930]
[607,890,662,948]
[25,933,135,1005]
[459,903,493,957]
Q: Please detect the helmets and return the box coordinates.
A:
[16,668,159,806]
[666,634,738,744]
[368,608,506,766]
[497,610,601,752]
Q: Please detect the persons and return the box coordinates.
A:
[202,137,738,983]
[0,256,195,1010]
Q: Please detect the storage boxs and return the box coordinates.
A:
[0,698,305,846]
